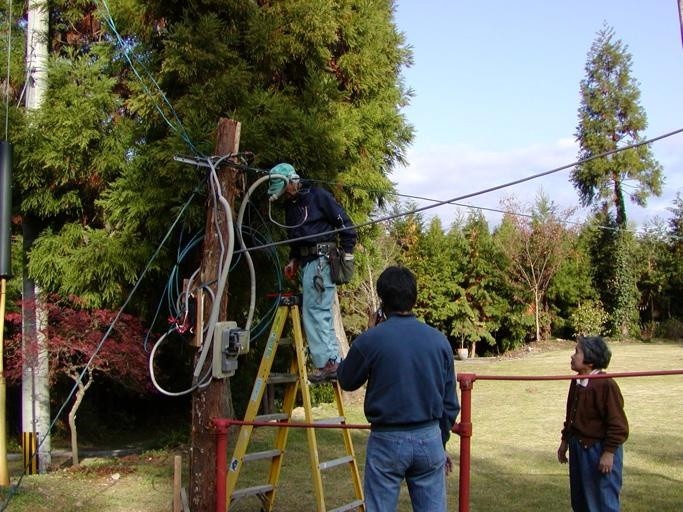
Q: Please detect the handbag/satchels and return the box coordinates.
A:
[326,245,355,287]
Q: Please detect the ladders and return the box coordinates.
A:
[213,295,369,512]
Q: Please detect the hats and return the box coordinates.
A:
[266,162,296,194]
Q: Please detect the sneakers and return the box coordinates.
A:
[306,360,340,383]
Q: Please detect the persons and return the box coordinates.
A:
[335,266,461,512]
[558,335,629,512]
[267,163,358,382]
